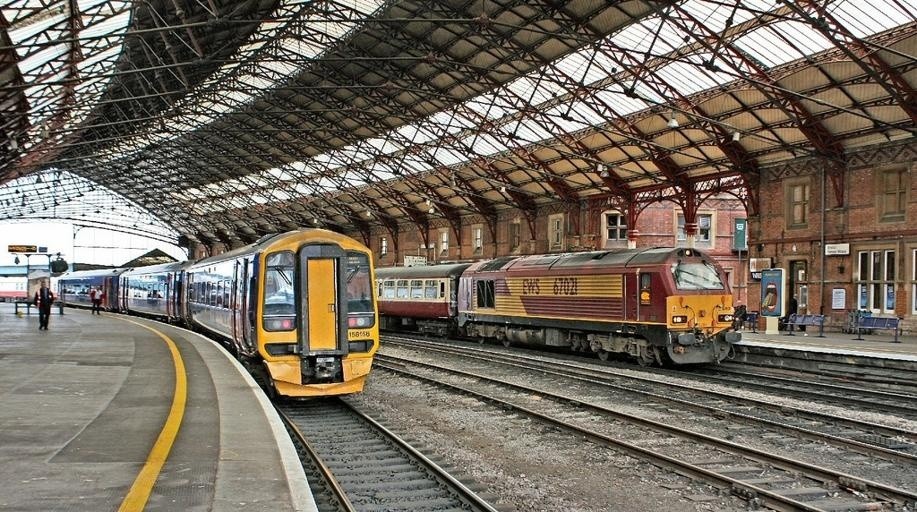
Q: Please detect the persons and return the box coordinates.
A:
[34,281,54,330]
[732,300,747,328]
[91,286,104,315]
[790,294,799,331]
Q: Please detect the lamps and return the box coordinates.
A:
[501,186,506,193]
[426,198,430,205]
[366,210,371,217]
[733,129,740,142]
[429,208,434,213]
[600,166,609,178]
[313,217,318,224]
[596,163,603,171]
[666,111,678,127]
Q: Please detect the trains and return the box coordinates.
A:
[376,247,748,369]
[1,228,379,405]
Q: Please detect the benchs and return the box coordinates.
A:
[783,313,826,337]
[852,315,901,343]
[744,312,758,332]
